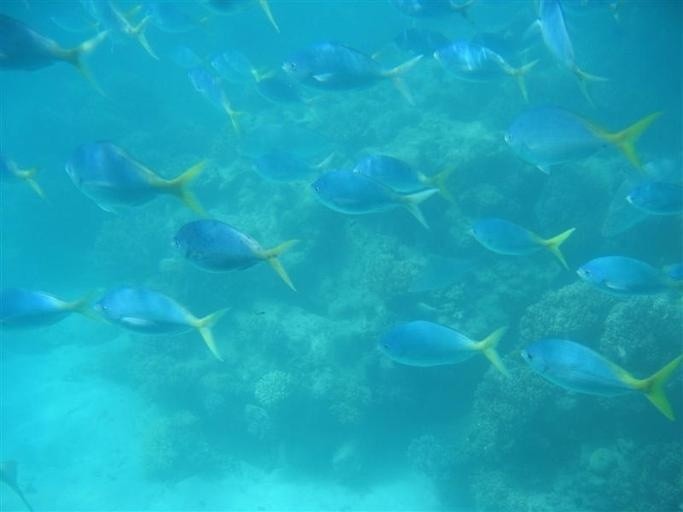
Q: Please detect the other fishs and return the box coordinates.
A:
[0,0,683,229]
